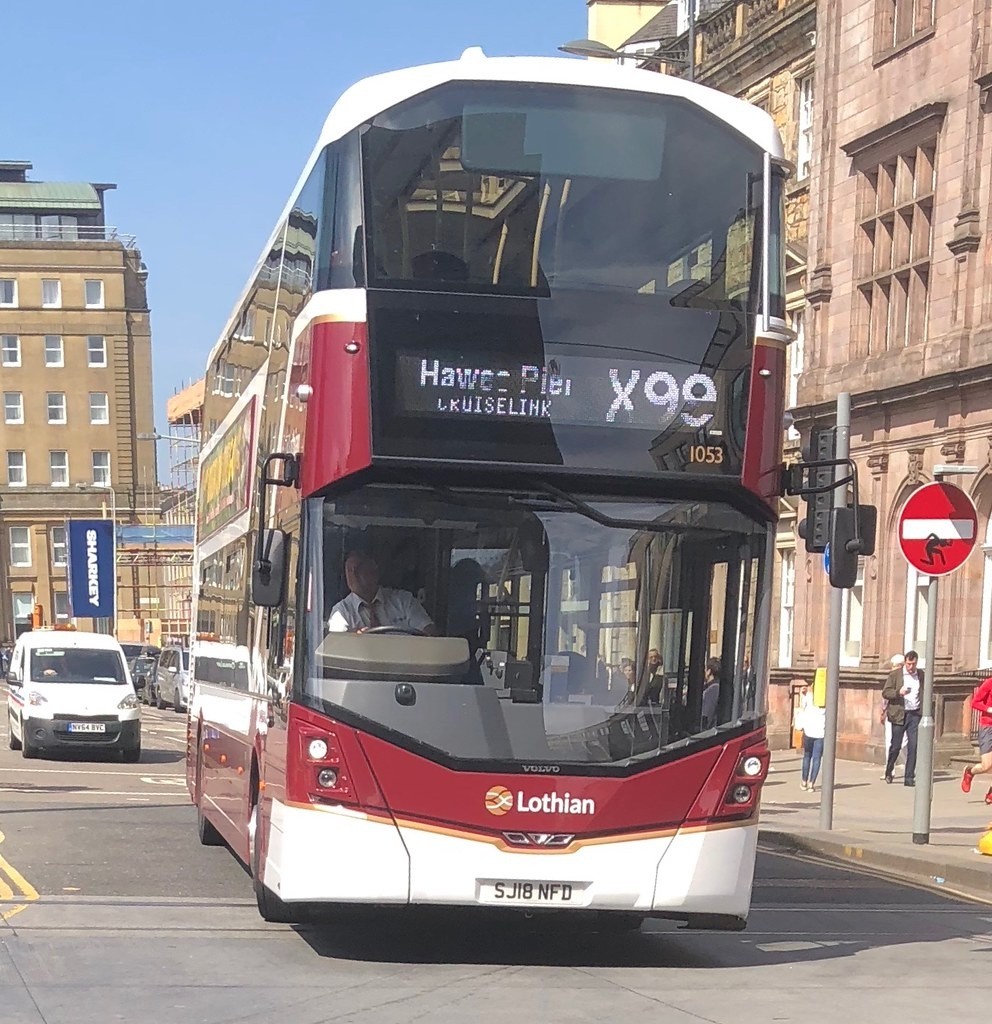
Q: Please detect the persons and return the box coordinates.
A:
[799,666,826,792]
[961,678,992,805]
[880,650,924,786]
[33,657,66,679]
[597,647,721,731]
[325,549,441,637]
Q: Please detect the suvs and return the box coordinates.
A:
[154,645,190,713]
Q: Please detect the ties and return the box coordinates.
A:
[369,603,386,634]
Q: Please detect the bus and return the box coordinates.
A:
[185,47,876,936]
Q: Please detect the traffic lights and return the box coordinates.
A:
[797,423,839,553]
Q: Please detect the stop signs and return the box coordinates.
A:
[896,482,980,576]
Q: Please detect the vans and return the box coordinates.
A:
[5,629,146,763]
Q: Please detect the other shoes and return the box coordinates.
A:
[880,772,895,780]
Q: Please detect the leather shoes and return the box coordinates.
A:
[885,771,892,784]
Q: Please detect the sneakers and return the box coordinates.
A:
[808,786,814,792]
[985,786,992,804]
[800,782,807,790]
[961,765,973,793]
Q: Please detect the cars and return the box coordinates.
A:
[120,642,157,707]
